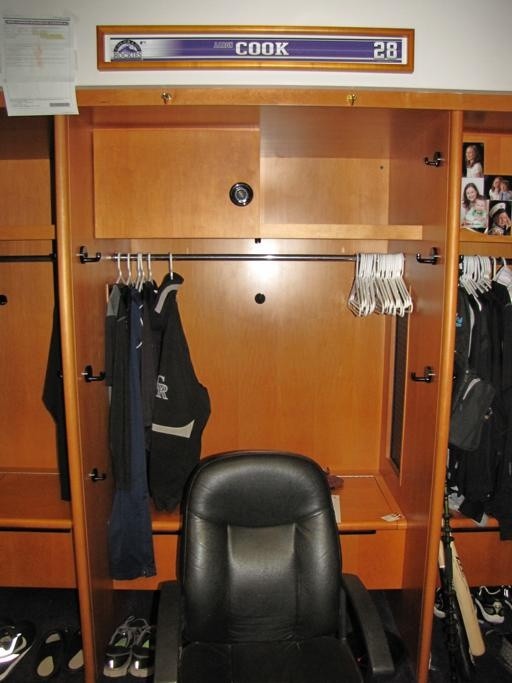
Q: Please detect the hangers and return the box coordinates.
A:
[458,256,507,312]
[348,251,416,319]
[104,252,174,303]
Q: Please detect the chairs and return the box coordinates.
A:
[154,450,396,682]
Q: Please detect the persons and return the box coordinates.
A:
[463,143,483,178]
[488,175,503,200]
[460,182,483,213]
[497,179,512,201]
[463,194,489,229]
[487,201,511,234]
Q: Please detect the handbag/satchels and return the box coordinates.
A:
[448,370,497,452]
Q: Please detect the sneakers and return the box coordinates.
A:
[102,616,157,678]
[0,622,37,682]
[434,583,511,623]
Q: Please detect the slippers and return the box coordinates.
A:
[37,628,85,678]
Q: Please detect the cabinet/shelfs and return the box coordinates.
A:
[0,85,512,683]
